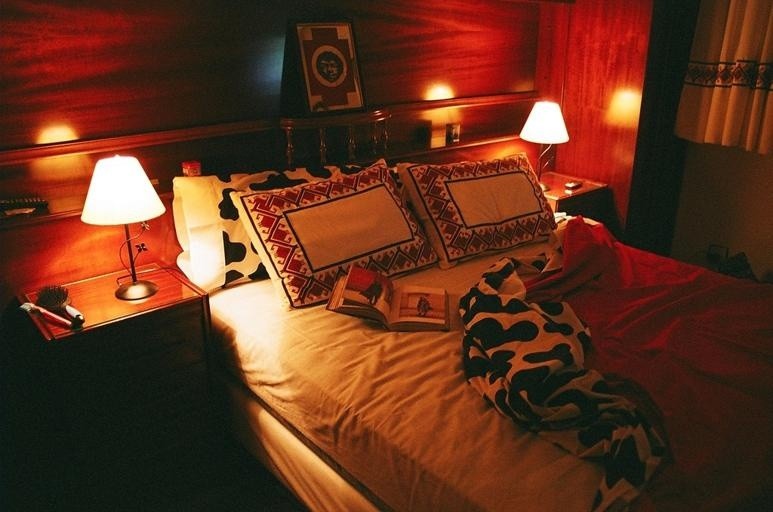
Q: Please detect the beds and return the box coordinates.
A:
[160,207,771,512]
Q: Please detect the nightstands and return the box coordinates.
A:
[537,171,610,229]
[15,260,237,510]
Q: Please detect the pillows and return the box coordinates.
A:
[174,163,404,294]
[396,154,559,270]
[229,157,439,313]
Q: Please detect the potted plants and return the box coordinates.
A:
[291,16,365,120]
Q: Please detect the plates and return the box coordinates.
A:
[533,2,700,258]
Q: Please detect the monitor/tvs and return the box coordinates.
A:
[327,263,450,335]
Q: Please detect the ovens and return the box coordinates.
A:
[160,207,771,512]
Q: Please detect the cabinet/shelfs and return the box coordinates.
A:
[533,2,700,258]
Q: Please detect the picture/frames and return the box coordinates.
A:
[291,16,365,120]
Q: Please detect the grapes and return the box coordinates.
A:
[537,171,610,229]
[15,260,237,510]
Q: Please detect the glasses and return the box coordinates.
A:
[77,153,167,305]
[518,99,569,192]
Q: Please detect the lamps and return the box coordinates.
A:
[77,153,167,305]
[518,99,569,192]
